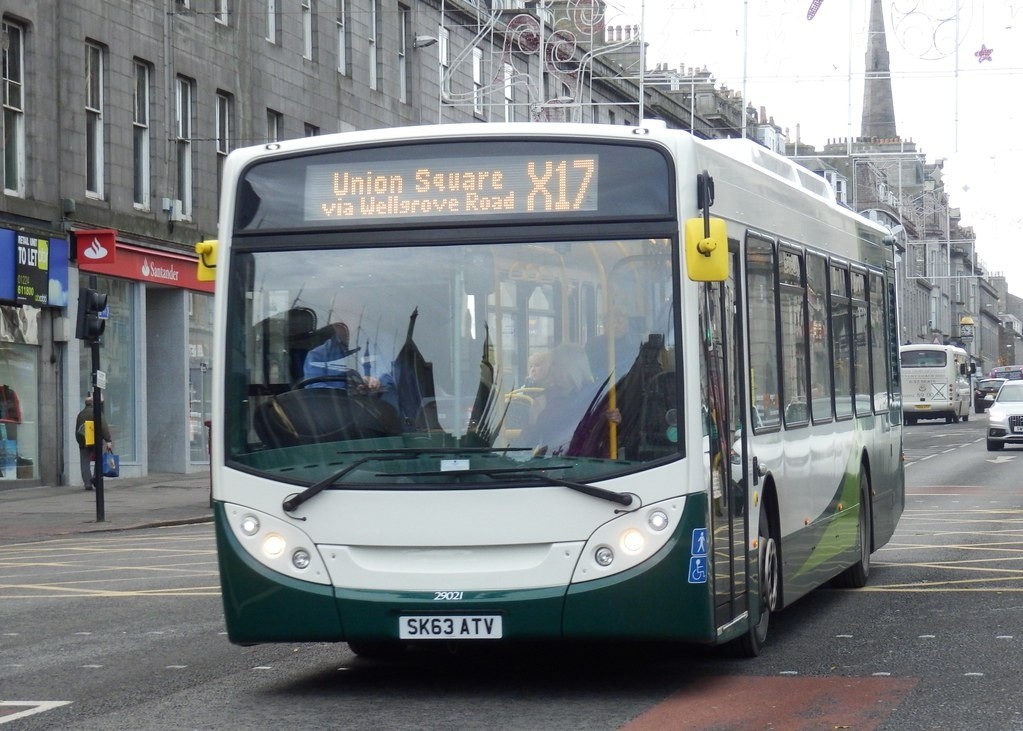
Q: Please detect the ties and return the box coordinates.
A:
[344,347,354,393]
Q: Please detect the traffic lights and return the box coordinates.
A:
[75,286,108,342]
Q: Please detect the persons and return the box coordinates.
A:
[303,291,401,414]
[76,398,112,491]
[522,307,634,458]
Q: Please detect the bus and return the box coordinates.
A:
[192,116,908,663]
[899,340,977,426]
[987,365,1023,380]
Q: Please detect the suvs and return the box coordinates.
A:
[974,378,1010,413]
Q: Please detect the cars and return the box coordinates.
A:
[983,377,1023,452]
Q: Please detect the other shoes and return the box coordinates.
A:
[90,476,96,487]
[85,482,92,490]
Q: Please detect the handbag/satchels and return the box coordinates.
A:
[103,448,119,477]
